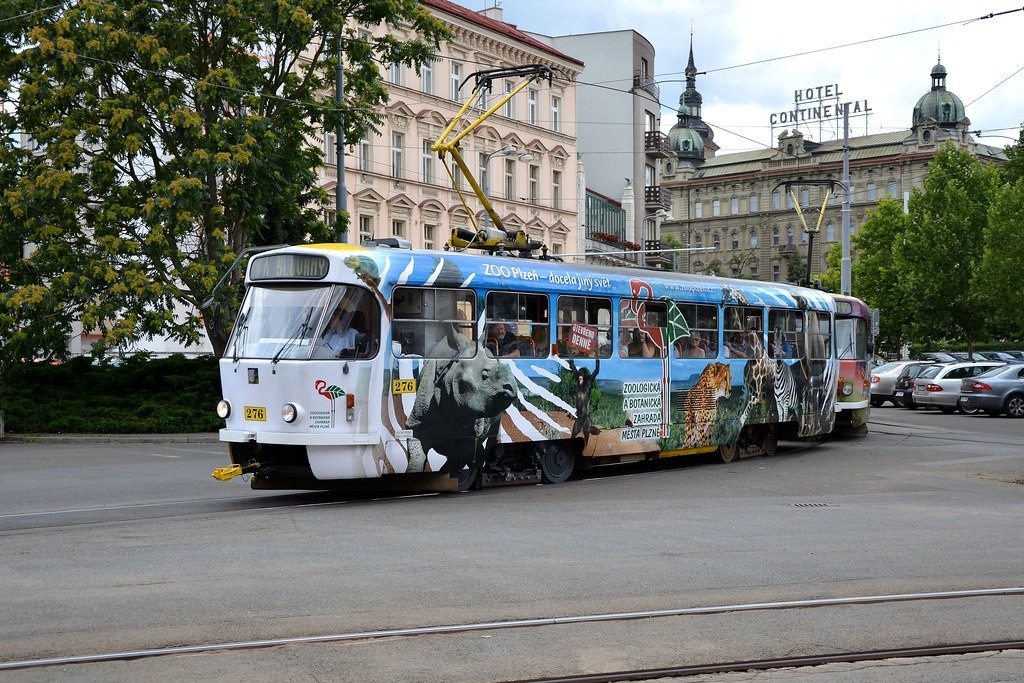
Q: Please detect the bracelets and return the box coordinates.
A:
[642,341,646,344]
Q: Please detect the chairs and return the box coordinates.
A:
[487,336,708,361]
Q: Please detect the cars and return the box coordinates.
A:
[868,350,1024,417]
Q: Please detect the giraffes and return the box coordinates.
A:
[720,286,776,426]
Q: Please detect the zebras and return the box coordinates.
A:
[772,324,799,423]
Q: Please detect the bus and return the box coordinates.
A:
[193,64,880,502]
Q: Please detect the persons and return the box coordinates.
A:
[487,319,520,357]
[706,332,729,358]
[682,333,705,358]
[535,327,548,355]
[674,347,680,358]
[628,328,655,358]
[774,327,792,358]
[599,330,628,357]
[726,330,753,358]
[323,308,358,349]
